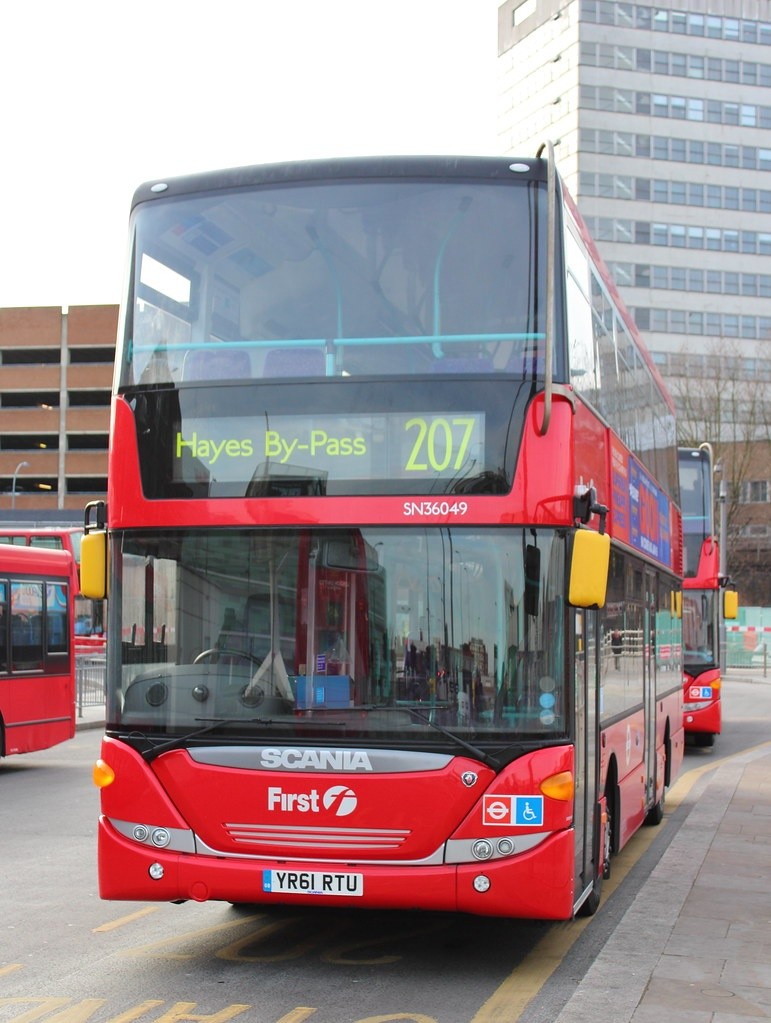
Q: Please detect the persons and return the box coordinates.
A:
[611,629,623,670]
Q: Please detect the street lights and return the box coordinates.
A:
[11,461,30,508]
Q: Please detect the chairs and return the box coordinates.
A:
[181,344,557,376]
[18,612,63,645]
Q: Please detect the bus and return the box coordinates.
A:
[79,139,684,920]
[0,525,95,758]
[678,442,740,746]
